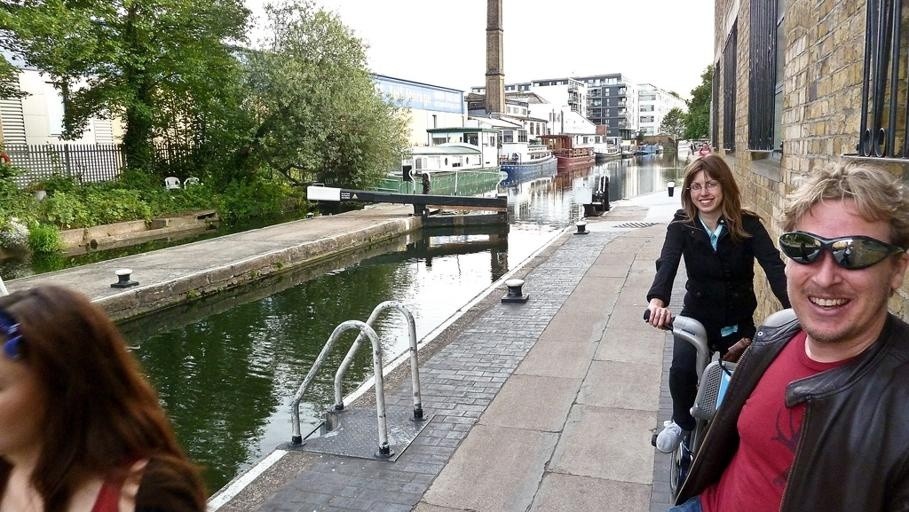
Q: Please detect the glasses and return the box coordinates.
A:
[778,231,905,270]
[686,180,720,192]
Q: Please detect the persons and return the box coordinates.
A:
[667,159,909,512]
[647,155,792,455]
[0,286,205,512]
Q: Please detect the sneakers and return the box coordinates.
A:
[656,419,689,453]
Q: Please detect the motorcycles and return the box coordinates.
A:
[643,280,757,501]
